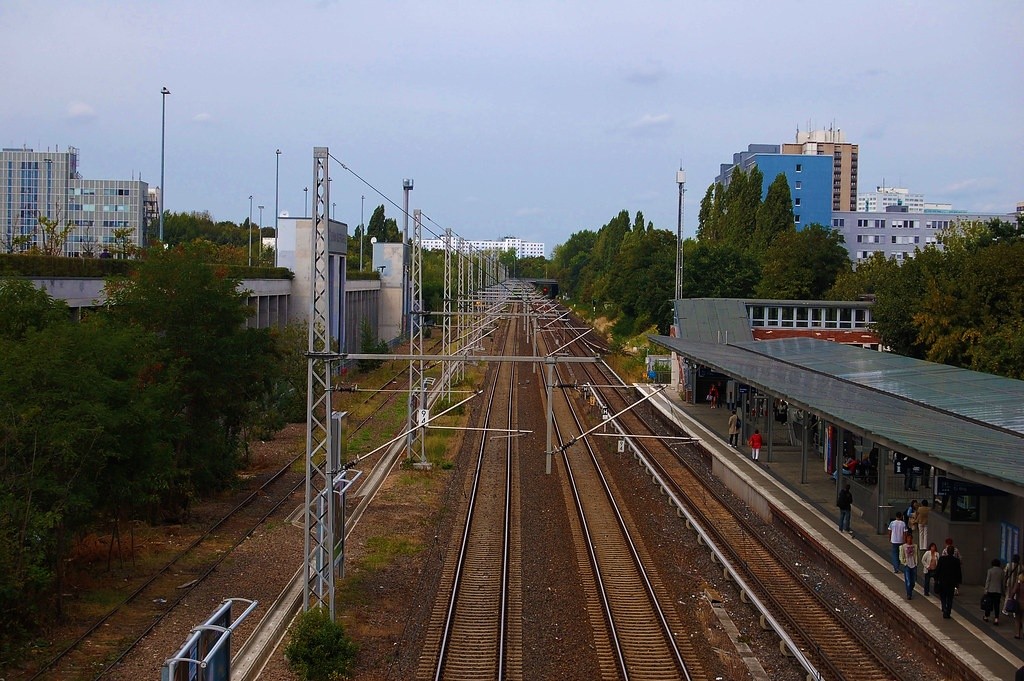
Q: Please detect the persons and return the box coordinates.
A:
[888,512,908,573]
[941,537,962,597]
[899,533,921,599]
[922,543,940,596]
[839,485,855,533]
[1001,554,1024,618]
[906,499,920,540]
[708,381,932,491]
[916,500,930,551]
[983,558,1006,625]
[1009,562,1024,639]
[936,545,961,619]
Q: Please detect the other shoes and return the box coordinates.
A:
[1002,610,1009,615]
[907,594,912,599]
[1012,612,1016,618]
[953,588,959,596]
[993,622,999,626]
[983,615,989,622]
[943,609,952,619]
[1015,634,1022,639]
[924,592,929,594]
[894,570,899,573]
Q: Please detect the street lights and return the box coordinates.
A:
[275,149,282,266]
[360,195,365,271]
[403,178,413,342]
[303,187,308,218]
[258,206,264,266]
[159,86,171,243]
[248,196,254,266]
[332,203,336,219]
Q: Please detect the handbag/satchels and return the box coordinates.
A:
[1005,576,1010,583]
[706,391,716,400]
[736,416,741,430]
[1005,599,1019,613]
[980,593,993,612]
